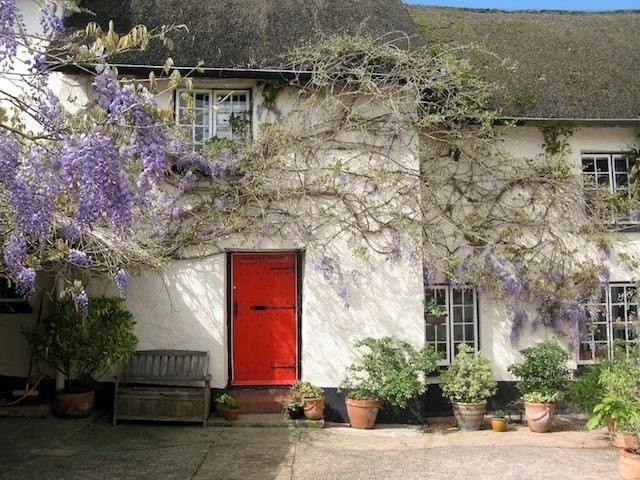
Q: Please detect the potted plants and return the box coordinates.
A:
[585,359,640,480]
[280,381,326,420]
[214,391,240,420]
[438,340,575,433]
[336,335,448,429]
[17,295,139,421]
[422,296,448,325]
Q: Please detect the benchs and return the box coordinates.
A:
[112,349,213,428]
